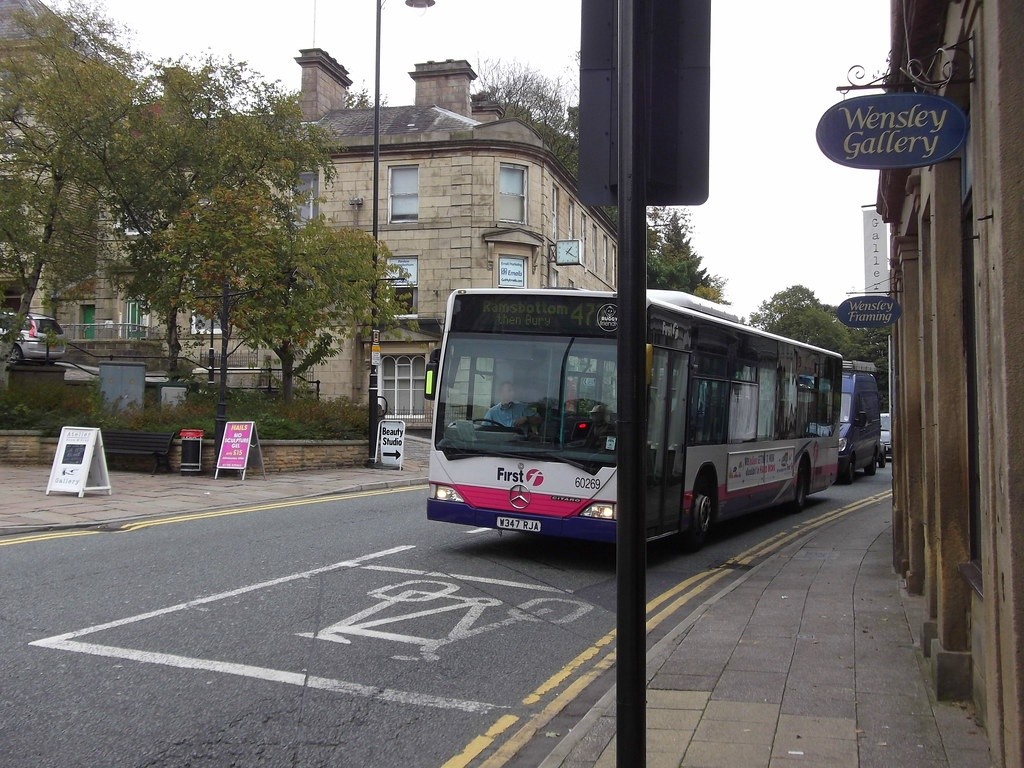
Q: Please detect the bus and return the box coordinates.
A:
[423,288,843,554]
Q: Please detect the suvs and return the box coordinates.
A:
[879,412,892,461]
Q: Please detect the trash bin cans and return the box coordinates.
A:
[181,429,204,476]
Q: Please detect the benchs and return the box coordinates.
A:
[102,430,176,475]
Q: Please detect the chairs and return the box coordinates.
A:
[519,388,832,454]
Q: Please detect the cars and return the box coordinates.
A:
[0,312,66,366]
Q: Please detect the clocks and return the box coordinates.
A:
[555,239,582,265]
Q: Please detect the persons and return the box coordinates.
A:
[590,405,616,451]
[482,381,542,436]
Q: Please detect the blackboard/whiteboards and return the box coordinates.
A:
[48,427,111,492]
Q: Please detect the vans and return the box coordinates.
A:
[836,360,887,484]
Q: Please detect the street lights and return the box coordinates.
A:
[363,0,435,473]
